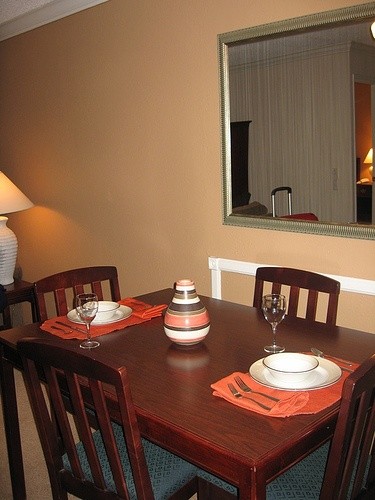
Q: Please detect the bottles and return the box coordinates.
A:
[163,279,210,346]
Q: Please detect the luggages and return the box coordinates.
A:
[272,187,317,220]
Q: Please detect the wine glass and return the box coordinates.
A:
[75,293,100,349]
[262,293,288,353]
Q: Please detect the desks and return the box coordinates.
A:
[0,288,375,500]
[0,280,39,330]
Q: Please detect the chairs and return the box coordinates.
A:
[253,266,341,326]
[196,353,375,500]
[33,265,122,322]
[17,337,197,500]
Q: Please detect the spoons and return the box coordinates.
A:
[50,325,73,334]
[311,348,353,366]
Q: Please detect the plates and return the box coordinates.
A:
[66,306,133,326]
[249,355,343,392]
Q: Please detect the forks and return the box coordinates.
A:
[228,383,272,411]
[234,375,280,403]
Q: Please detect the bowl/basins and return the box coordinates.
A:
[82,300,120,322]
[262,352,320,383]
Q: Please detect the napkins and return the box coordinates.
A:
[210,371,309,418]
[117,297,168,319]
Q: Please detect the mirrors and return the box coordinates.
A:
[216,2,375,241]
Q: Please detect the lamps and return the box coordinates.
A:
[363,147,373,179]
[0,170,36,286]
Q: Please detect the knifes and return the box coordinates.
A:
[56,321,87,334]
